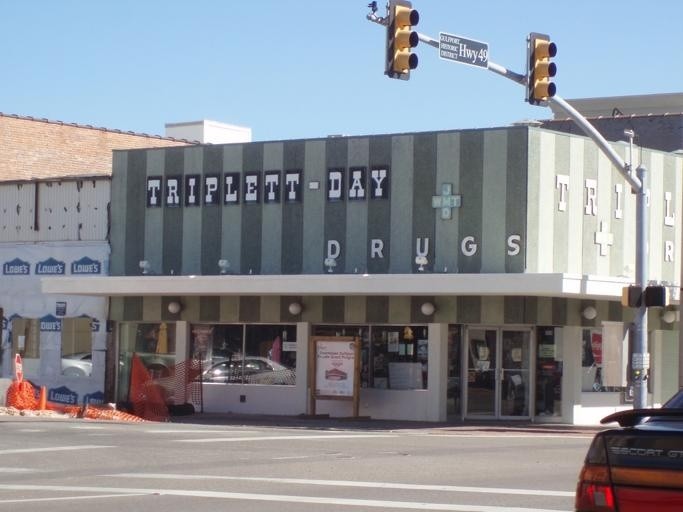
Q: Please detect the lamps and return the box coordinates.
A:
[288,302,302,315]
[583,305,597,320]
[167,301,182,314]
[421,302,435,316]
[662,309,675,323]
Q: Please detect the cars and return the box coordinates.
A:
[451,367,553,416]
[60,351,172,379]
[191,356,291,385]
[574,389,682,512]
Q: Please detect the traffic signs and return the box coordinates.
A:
[438,31,488,70]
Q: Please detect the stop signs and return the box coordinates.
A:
[13,354,23,382]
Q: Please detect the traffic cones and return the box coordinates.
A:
[38,382,48,411]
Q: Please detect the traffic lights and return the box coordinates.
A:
[390,4,418,73]
[645,285,669,307]
[620,286,641,308]
[532,38,557,102]
